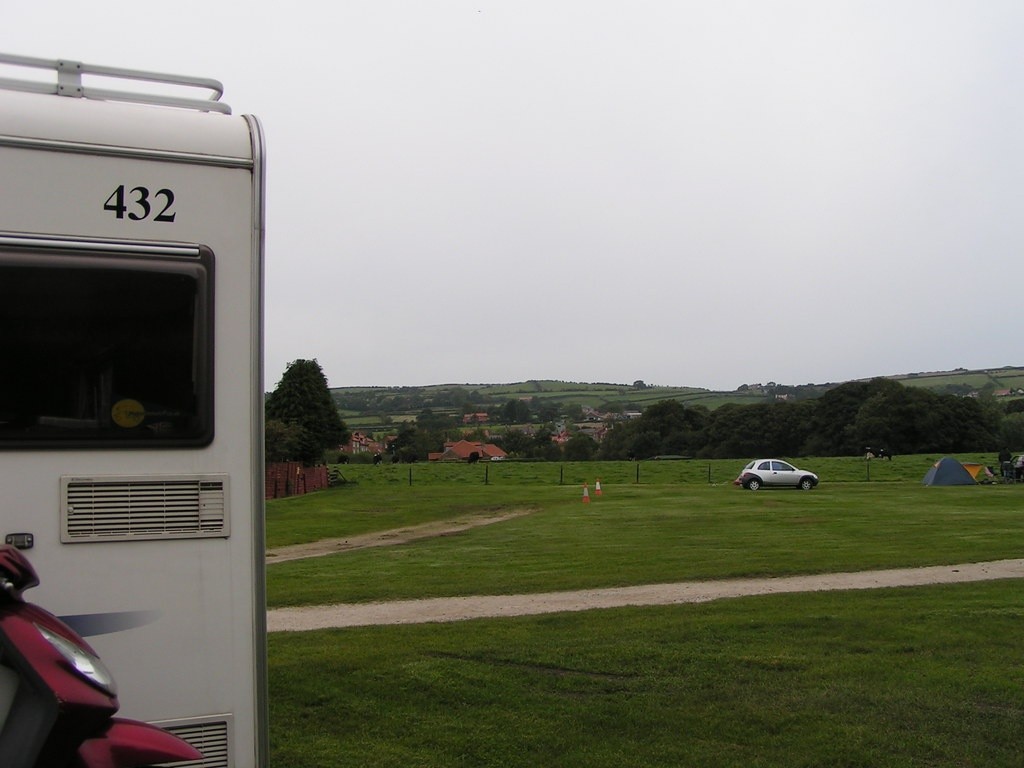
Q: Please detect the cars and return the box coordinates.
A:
[733,458,819,491]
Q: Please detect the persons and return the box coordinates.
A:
[880,448,892,460]
[865,445,871,460]
[999,445,1012,476]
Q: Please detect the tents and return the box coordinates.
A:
[923,457,983,485]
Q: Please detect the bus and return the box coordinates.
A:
[0,51,267,767]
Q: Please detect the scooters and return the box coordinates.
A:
[1,537,208,768]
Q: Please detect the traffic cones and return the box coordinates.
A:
[580,482,590,503]
[593,478,600,496]
